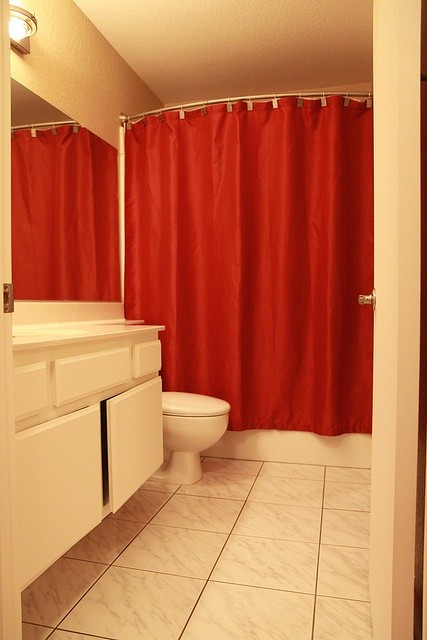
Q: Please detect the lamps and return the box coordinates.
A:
[8,4,37,45]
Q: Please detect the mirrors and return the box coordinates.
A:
[12,78,126,304]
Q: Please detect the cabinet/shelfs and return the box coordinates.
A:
[11,326,163,590]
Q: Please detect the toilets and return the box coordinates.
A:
[149,388,230,486]
